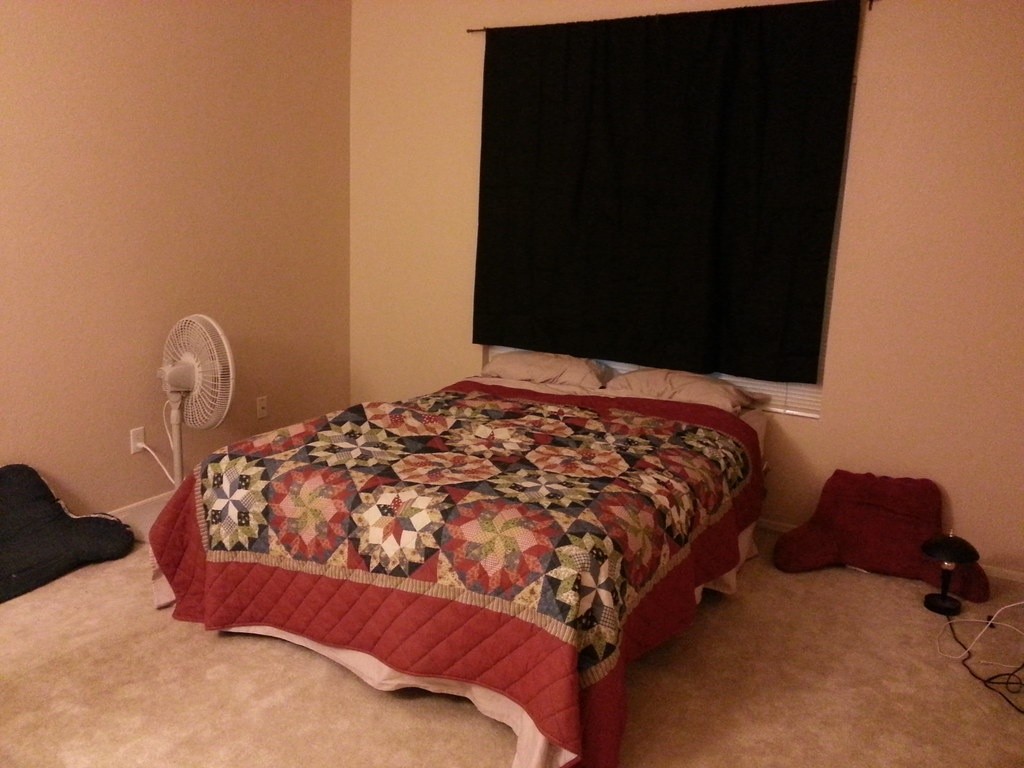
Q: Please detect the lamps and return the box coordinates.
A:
[920,529,980,617]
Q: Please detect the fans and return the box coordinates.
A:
[158,316,236,488]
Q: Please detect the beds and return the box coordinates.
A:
[149,381,772,768]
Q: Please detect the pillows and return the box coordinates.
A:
[480,351,619,390]
[609,369,762,414]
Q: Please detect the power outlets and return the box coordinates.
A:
[258,396,267,418]
[129,427,144,454]
